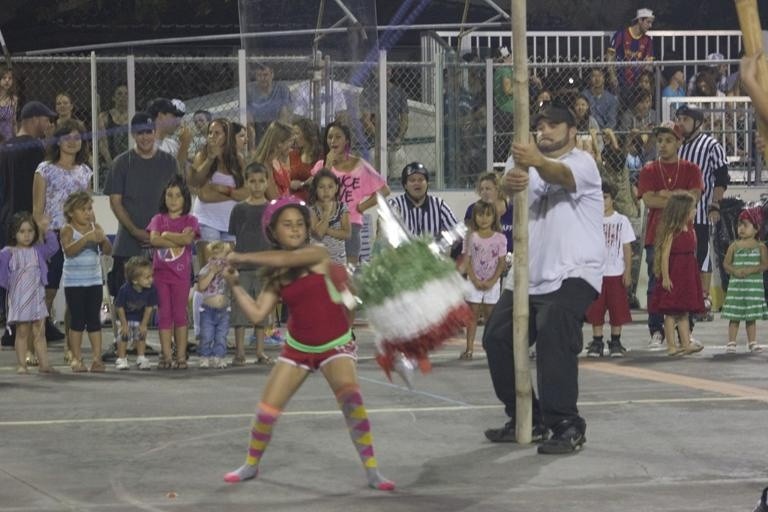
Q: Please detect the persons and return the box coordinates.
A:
[576,104,768,358]
[220,194,397,490]
[1,63,408,373]
[444,8,768,184]
[377,161,513,360]
[482,102,607,455]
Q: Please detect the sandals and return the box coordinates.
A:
[726,341,736,353]
[749,341,762,353]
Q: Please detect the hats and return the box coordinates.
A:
[21,101,57,118]
[652,121,683,139]
[530,103,575,128]
[706,53,724,67]
[151,99,185,116]
[493,45,510,63]
[632,8,655,21]
[740,207,762,225]
[131,112,154,126]
[662,66,682,80]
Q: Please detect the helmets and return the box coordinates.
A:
[401,163,429,185]
[261,195,312,248]
[676,104,704,121]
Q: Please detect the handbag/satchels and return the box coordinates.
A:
[700,270,725,312]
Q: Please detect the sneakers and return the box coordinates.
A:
[649,328,704,356]
[461,351,472,360]
[586,340,604,357]
[46,317,65,340]
[17,353,59,374]
[1,324,15,345]
[607,339,626,357]
[538,418,585,454]
[528,347,536,361]
[65,329,284,372]
[485,417,549,442]
